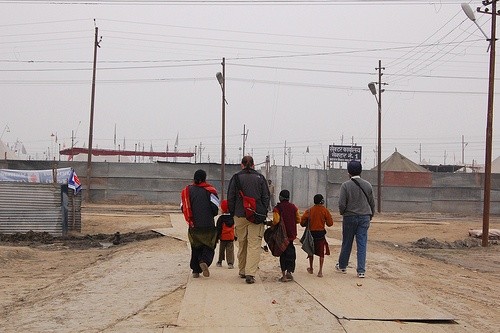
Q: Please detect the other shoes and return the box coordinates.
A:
[317,273,323,277]
[216,261,222,266]
[307,268,313,274]
[193,273,198,277]
[279,277,288,282]
[228,264,233,269]
[246,276,256,283]
[241,275,245,278]
[200,263,209,277]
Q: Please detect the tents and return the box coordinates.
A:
[371,148,434,171]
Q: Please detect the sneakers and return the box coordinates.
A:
[357,273,365,278]
[335,263,346,274]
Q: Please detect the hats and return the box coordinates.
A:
[348,160,361,175]
[221,200,228,212]
[314,194,324,204]
[279,189,290,200]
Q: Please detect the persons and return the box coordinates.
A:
[300,194,333,277]
[267,190,301,282]
[227,156,270,284]
[216,199,238,269]
[180,169,219,278]
[335,161,375,278]
[267,179,275,212]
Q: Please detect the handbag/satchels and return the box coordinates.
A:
[264,216,289,257]
[299,227,314,258]
[241,194,268,224]
